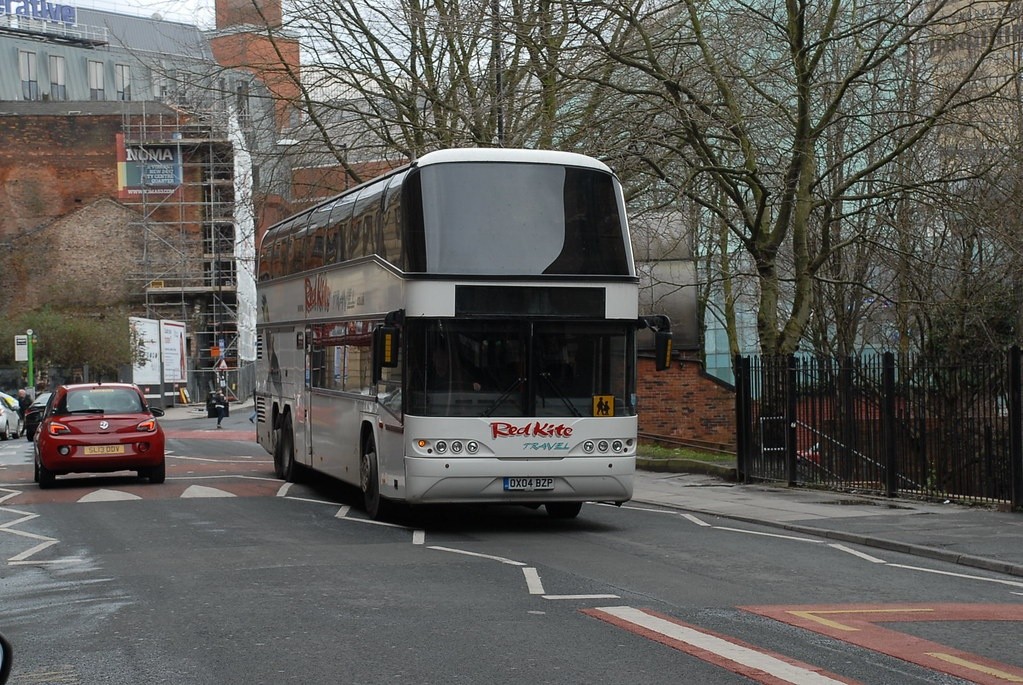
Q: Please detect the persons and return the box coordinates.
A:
[248,387,257,423]
[18,389,33,437]
[214,388,227,429]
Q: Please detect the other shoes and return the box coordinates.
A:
[249,418,254,423]
[217,426,224,428]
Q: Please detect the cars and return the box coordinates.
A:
[24,392,53,442]
[0,396,20,441]
[33,382,167,489]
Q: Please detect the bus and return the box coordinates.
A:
[254,146,673,520]
